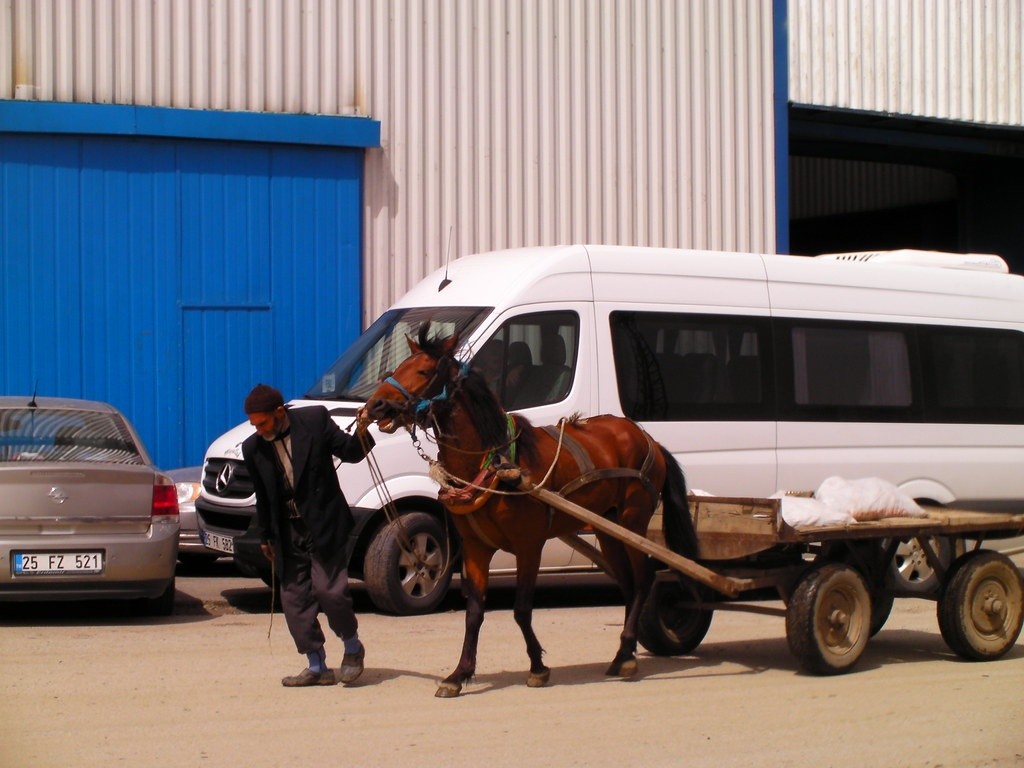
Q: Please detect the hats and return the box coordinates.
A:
[245,384,284,415]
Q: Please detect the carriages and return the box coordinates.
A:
[349,319,1022,696]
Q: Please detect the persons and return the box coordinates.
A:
[242,382,377,688]
[468,339,533,412]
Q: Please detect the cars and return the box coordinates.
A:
[0,389,185,621]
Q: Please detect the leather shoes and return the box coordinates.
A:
[282,641,365,686]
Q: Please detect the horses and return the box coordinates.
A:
[364,319,700,697]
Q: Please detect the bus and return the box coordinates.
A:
[189,232,1024,585]
